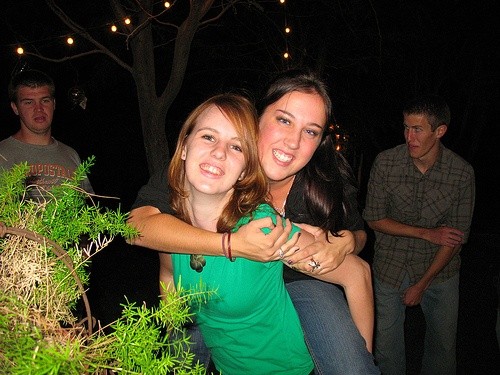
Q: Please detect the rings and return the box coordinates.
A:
[279,248,285,258]
[308,259,321,272]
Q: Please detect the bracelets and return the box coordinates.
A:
[222,231,237,261]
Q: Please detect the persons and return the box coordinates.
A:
[125,74,385,374]
[0,69,96,204]
[159,91,375,375]
[363,95,475,375]
